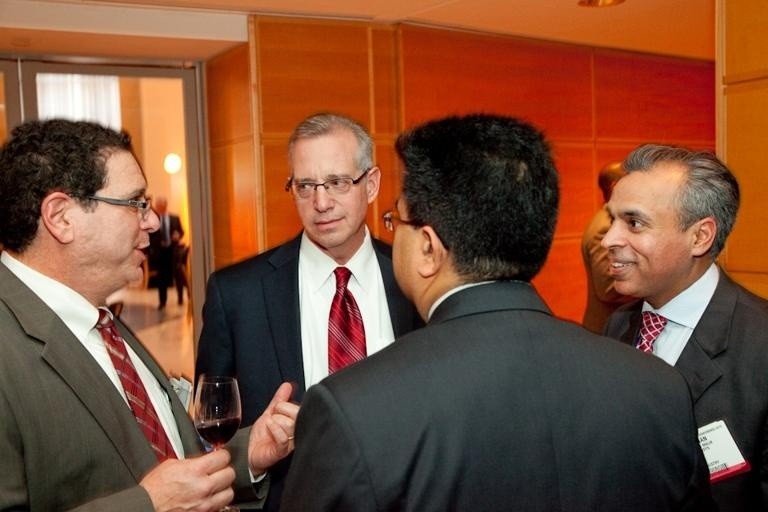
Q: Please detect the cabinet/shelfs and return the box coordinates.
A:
[195,13,718,325]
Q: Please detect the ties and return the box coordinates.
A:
[95,309,179,465]
[162,214,166,242]
[634,311,667,355]
[328,268,368,375]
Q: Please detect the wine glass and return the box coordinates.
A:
[194,374,245,511]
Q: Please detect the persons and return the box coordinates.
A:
[583,160,640,335]
[191,114,428,511]
[1,120,302,511]
[601,143,767,512]
[149,197,185,310]
[278,112,712,511]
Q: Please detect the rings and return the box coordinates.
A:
[287,435,297,444]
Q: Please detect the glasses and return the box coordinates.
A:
[66,193,151,222]
[285,168,373,201]
[381,209,448,251]
